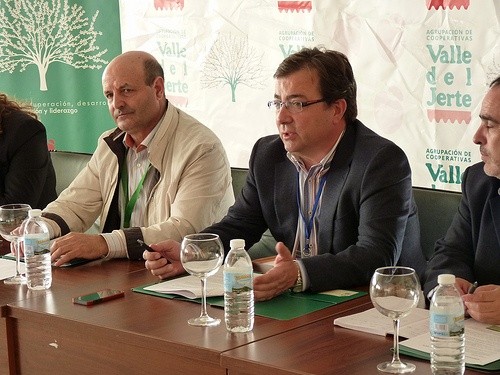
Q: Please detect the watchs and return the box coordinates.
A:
[290,267,302,293]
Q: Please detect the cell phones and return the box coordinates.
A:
[72,288,124,306]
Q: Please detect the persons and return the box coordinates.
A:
[0,93,58,212]
[10,51,234,267]
[144,46,426,299]
[424,77,500,325]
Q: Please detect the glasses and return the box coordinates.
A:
[267,98,325,112]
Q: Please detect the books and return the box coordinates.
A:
[333,306,431,339]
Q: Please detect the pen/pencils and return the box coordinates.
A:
[136,239,172,264]
[467,281,478,294]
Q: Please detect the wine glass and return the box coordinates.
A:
[179,233,225,326]
[0,204,32,285]
[369,266,421,374]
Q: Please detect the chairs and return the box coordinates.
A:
[49,149,101,234]
[414,187,461,256]
[230,167,277,260]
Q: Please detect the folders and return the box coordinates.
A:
[131,266,369,321]
[389,317,500,370]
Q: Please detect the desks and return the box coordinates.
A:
[220,303,500,375]
[7,268,371,375]
[0,239,147,375]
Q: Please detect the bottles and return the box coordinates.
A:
[428,273,465,375]
[23,209,53,291]
[223,238,255,332]
